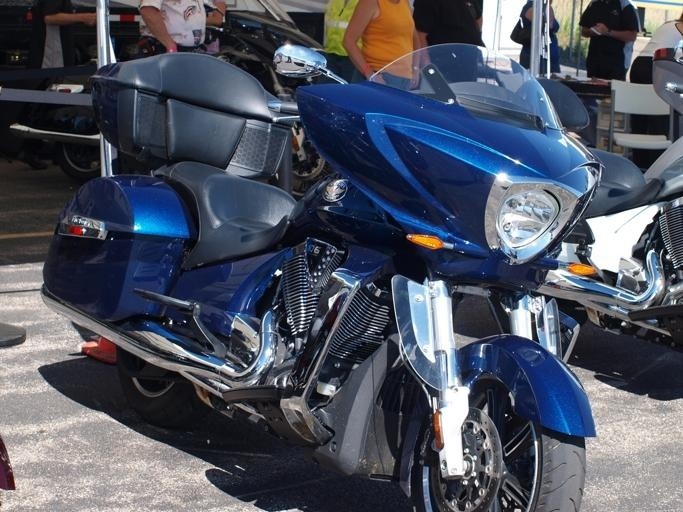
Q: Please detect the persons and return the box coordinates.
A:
[322,0,361,83]
[629,13,682,175]
[202,0,225,53]
[135,0,217,53]
[578,0,639,81]
[341,0,421,89]
[15,0,96,171]
[412,0,483,83]
[510,0,561,73]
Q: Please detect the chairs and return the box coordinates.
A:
[516,79,660,218]
[607,76,671,172]
[112,52,306,268]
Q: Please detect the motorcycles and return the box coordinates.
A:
[444,38,683,363]
[36,43,607,512]
[0,1,354,201]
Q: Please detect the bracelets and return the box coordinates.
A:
[360,65,372,75]
[606,28,610,36]
[412,65,418,71]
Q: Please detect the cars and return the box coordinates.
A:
[0,0,144,56]
